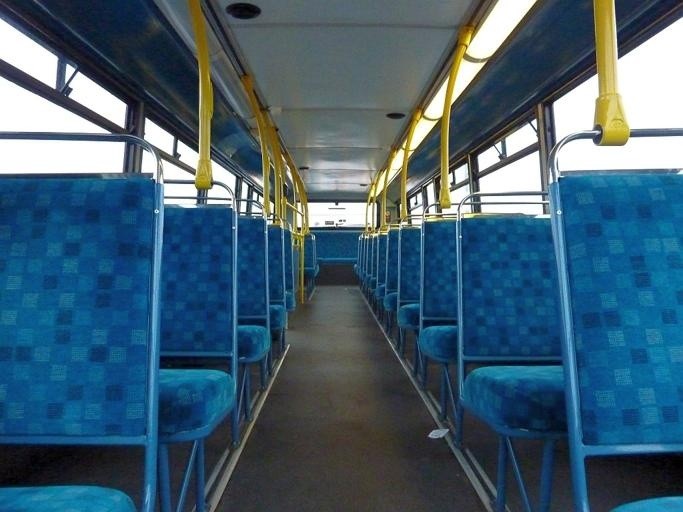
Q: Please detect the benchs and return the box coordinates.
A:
[296,226,372,285]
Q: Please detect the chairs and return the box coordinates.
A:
[354,127,683,512]
[0,131,321,512]
